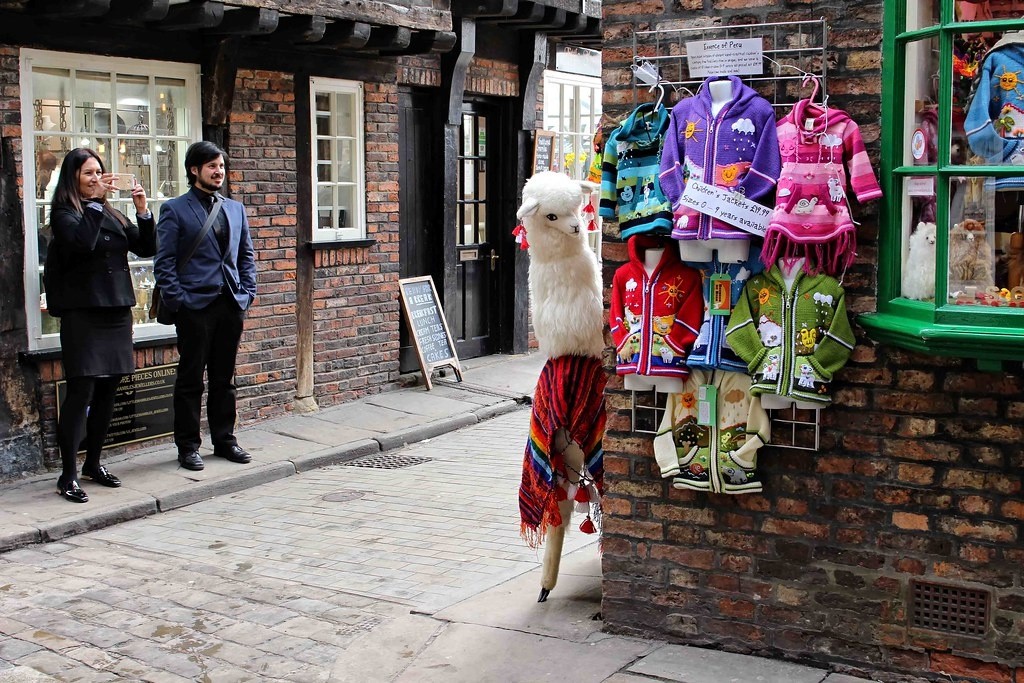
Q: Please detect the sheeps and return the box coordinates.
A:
[516,170,605,604]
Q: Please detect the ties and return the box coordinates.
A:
[205,195,228,256]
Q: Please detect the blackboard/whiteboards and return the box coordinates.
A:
[532,129,556,175]
[398,275,459,368]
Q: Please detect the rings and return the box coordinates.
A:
[103,183,110,188]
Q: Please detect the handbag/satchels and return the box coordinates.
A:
[44,238,92,322]
[148,281,175,326]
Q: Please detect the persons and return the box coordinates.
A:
[29,148,160,503]
[659,76,781,264]
[610,247,702,393]
[153,141,258,470]
[725,257,855,409]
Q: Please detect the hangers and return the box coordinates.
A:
[634,85,664,127]
[803,77,827,115]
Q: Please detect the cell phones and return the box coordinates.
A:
[112,173,134,190]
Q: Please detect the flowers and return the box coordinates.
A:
[564,151,587,181]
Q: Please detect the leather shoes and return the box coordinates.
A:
[178,451,204,470]
[56,475,88,503]
[81,463,122,488]
[213,444,252,464]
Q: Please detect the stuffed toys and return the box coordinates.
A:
[950,219,994,294]
[950,138,967,166]
[904,222,936,301]
